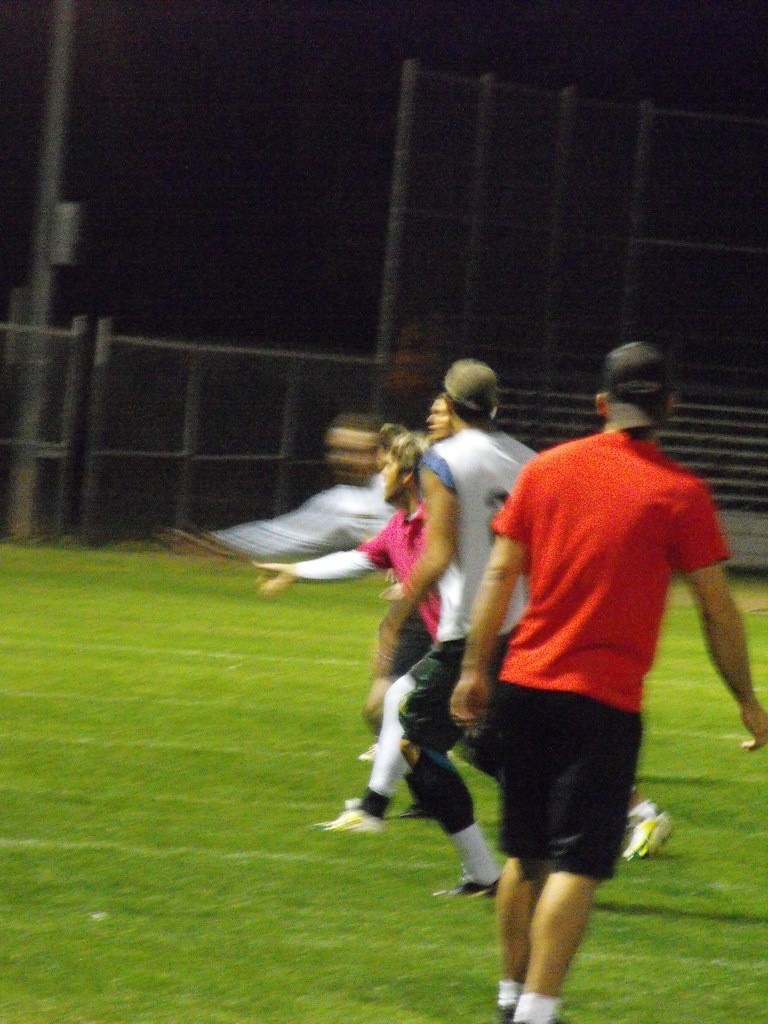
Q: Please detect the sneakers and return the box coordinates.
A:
[358,743,379,760]
[621,811,673,862]
[433,878,501,902]
[398,802,435,818]
[323,807,385,833]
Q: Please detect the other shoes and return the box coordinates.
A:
[495,1003,517,1023]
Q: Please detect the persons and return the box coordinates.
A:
[449,340,768,1024]
[157,358,674,900]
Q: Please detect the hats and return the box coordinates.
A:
[604,341,677,432]
[445,358,498,411]
